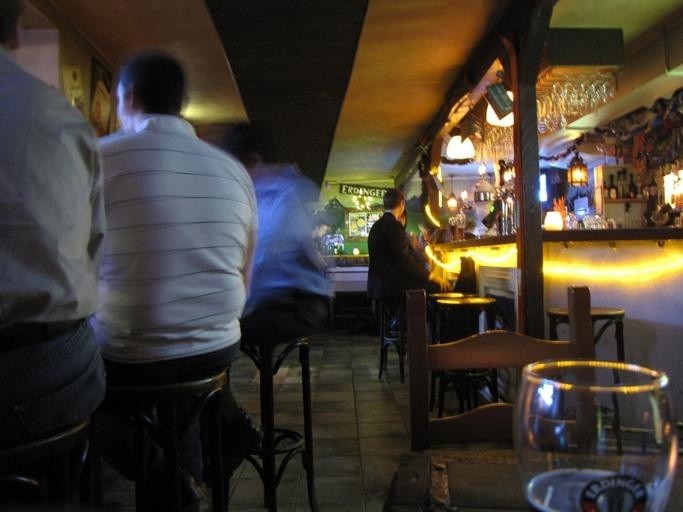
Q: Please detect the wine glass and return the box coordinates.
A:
[474,74,613,165]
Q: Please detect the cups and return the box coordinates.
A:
[510,358,678,512]
[447,212,465,242]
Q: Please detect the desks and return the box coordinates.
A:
[375,440,683,512]
[327,264,369,331]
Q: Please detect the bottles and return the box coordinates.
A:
[599,168,657,201]
[543,211,563,232]
[322,233,344,255]
[504,192,516,235]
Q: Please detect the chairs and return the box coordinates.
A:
[401,281,599,451]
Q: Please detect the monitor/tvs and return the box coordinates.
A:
[539,168,568,210]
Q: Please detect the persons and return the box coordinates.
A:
[0,0,108,512]
[311,219,329,239]
[198,123,335,488]
[396,210,408,229]
[366,188,452,344]
[90,53,259,512]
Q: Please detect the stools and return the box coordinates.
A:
[94,363,231,511]
[371,287,507,417]
[544,305,632,455]
[218,326,322,511]
[0,412,97,512]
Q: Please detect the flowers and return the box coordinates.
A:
[320,233,345,254]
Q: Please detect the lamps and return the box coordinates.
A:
[567,151,589,188]
[446,173,458,210]
[483,90,516,129]
[447,134,475,162]
